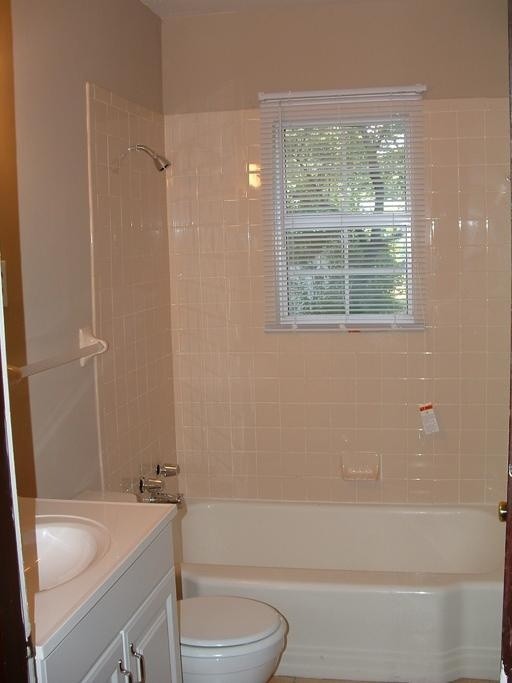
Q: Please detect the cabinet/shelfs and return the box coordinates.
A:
[33,504,184,682]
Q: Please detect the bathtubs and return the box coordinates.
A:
[172,497,507,683]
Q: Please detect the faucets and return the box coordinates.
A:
[150,493,183,505]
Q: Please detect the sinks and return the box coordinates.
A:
[35,521,97,592]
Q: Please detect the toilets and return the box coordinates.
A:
[66,489,289,683]
